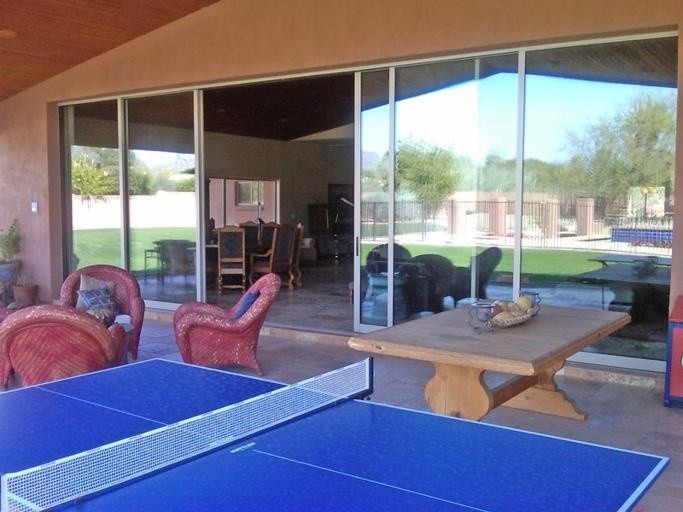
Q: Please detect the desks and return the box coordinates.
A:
[570,255,670,342]
[348,299,631,422]
[0,358,671,512]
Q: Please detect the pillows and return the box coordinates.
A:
[235,288,258,319]
[75,273,118,329]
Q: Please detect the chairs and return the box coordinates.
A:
[59,264,144,362]
[141,219,304,292]
[354,242,502,322]
[0,306,126,388]
[173,273,281,377]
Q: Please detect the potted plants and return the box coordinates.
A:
[11,268,40,305]
[0,221,23,305]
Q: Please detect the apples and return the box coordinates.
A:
[491,296,531,315]
